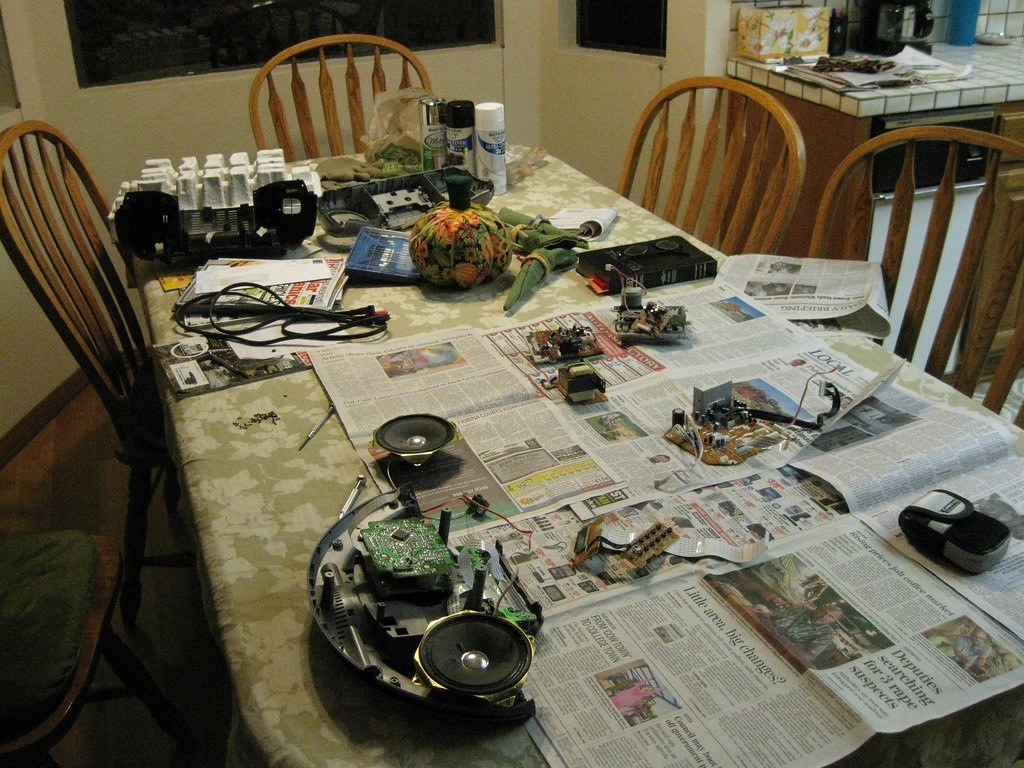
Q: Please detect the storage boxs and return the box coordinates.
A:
[737,6,829,63]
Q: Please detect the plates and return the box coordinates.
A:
[975,33,1018,45]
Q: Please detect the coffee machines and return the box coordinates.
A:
[852,0,934,57]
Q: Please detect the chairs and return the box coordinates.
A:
[0,121,171,628]
[0,527,129,762]
[616,75,806,257]
[247,33,431,164]
[810,123,1024,429]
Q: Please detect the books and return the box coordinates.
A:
[782,45,974,93]
[576,235,717,295]
[150,256,353,401]
[547,207,619,241]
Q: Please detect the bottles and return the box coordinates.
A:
[474,102,507,195]
[445,100,475,179]
[418,98,446,171]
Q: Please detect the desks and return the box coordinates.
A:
[112,145,1024,768]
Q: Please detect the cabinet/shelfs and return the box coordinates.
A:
[960,103,1024,358]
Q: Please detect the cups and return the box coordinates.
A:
[828,16,848,56]
[948,0,981,46]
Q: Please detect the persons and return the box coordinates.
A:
[953,628,991,667]
[762,283,790,295]
[647,454,670,465]
[600,413,626,427]
[766,603,844,663]
[608,676,667,716]
